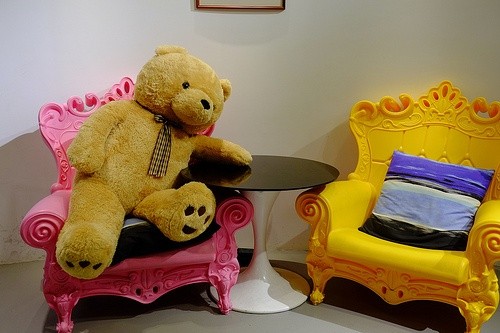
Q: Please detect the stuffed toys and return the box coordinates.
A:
[56,46,253,280]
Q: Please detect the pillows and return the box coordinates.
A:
[357,151,494,251]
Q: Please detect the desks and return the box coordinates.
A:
[180,154,339,315]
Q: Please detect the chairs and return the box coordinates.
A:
[296,81,500,333]
[20,77,255,333]
